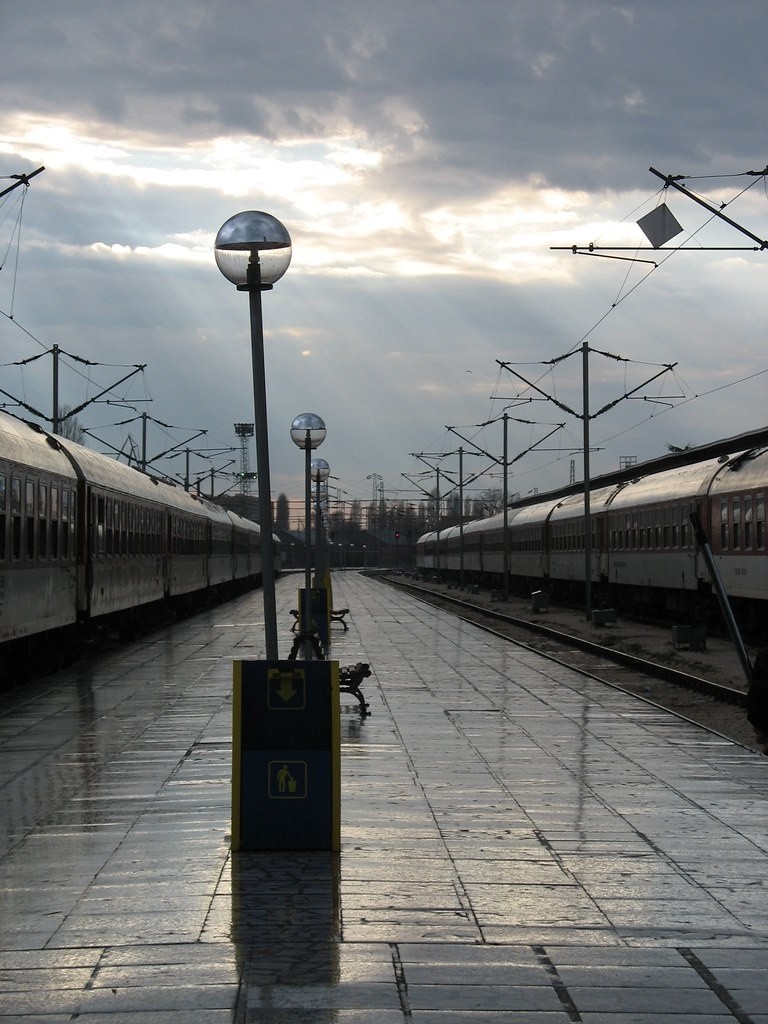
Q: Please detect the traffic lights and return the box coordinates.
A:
[394,531,400,540]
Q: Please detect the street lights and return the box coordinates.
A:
[290,412,328,662]
[338,543,342,568]
[362,544,368,569]
[310,457,331,587]
[350,544,354,567]
[212,209,293,663]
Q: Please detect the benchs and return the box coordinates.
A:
[329,608,350,631]
[289,609,299,632]
[288,632,303,660]
[306,629,372,717]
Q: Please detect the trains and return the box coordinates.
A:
[0,406,283,646]
[414,445,768,635]
